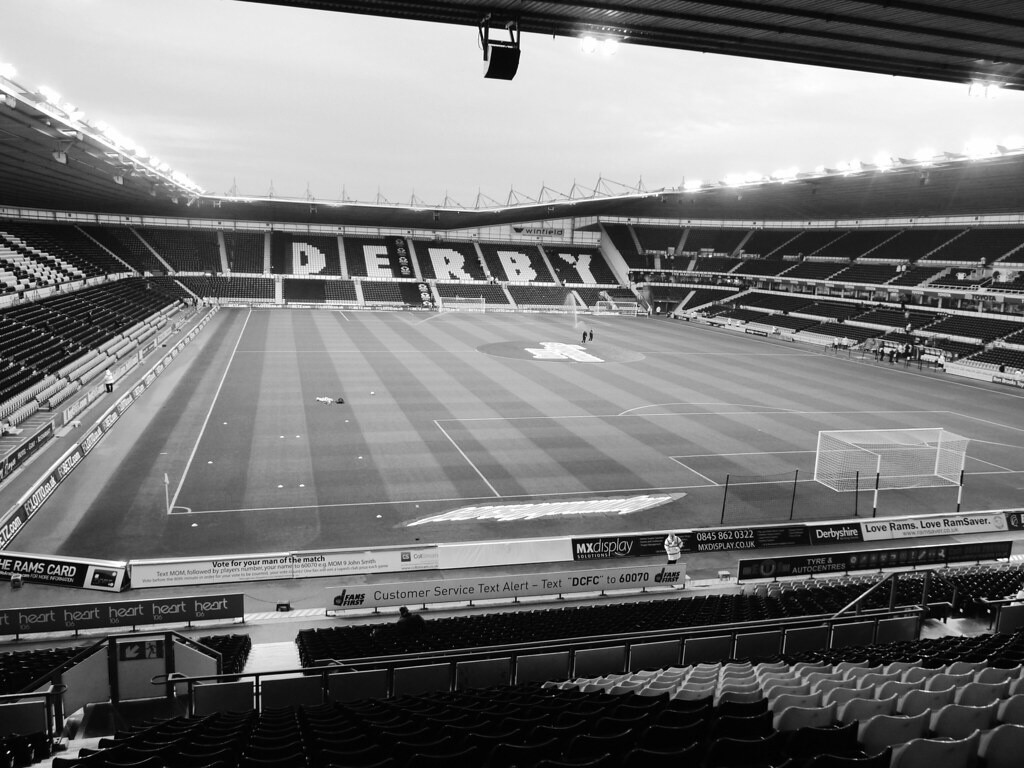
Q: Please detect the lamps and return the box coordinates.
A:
[479,14,520,80]
[53,139,75,164]
[113,166,124,184]
[919,171,929,185]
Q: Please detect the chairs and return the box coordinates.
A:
[0,560,1024,768]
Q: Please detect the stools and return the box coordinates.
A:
[11,574,24,588]
[276,601,291,611]
[718,571,730,581]
[685,574,690,584]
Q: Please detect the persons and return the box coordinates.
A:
[834,335,848,350]
[664,534,684,564]
[589,330,593,341]
[727,318,731,325]
[999,363,1005,372]
[656,307,661,315]
[872,343,910,363]
[582,330,587,343]
[1016,575,1024,603]
[103,368,114,392]
[490,275,498,286]
[735,319,740,327]
